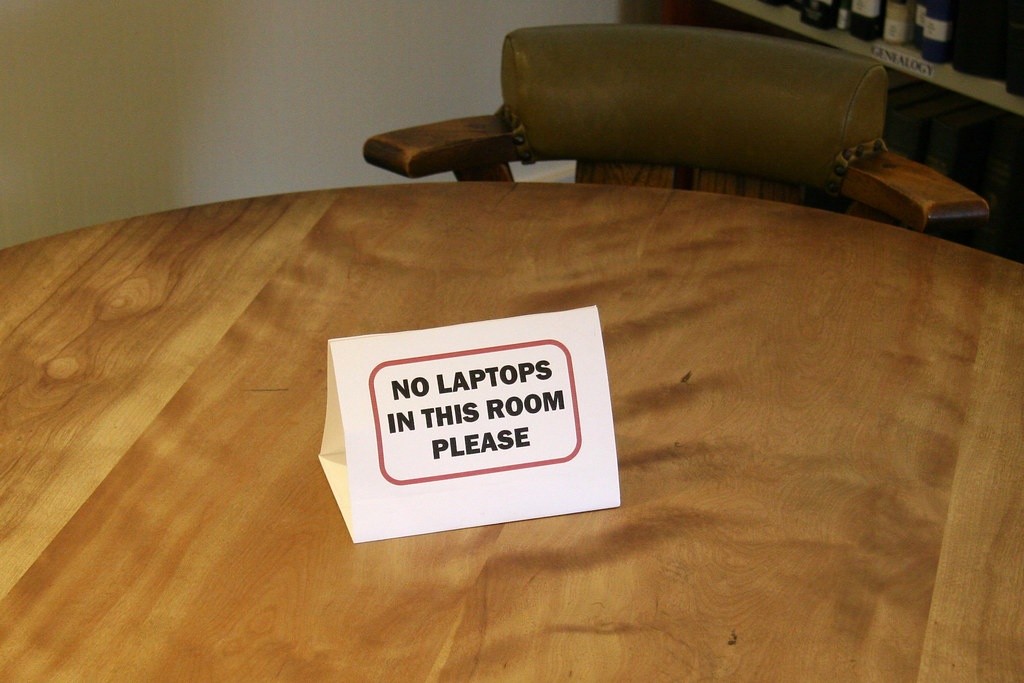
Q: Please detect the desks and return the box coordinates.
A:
[2,180,1024,682]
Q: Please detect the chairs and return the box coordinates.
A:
[363,25,989,250]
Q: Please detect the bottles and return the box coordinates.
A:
[766,0,1024,96]
